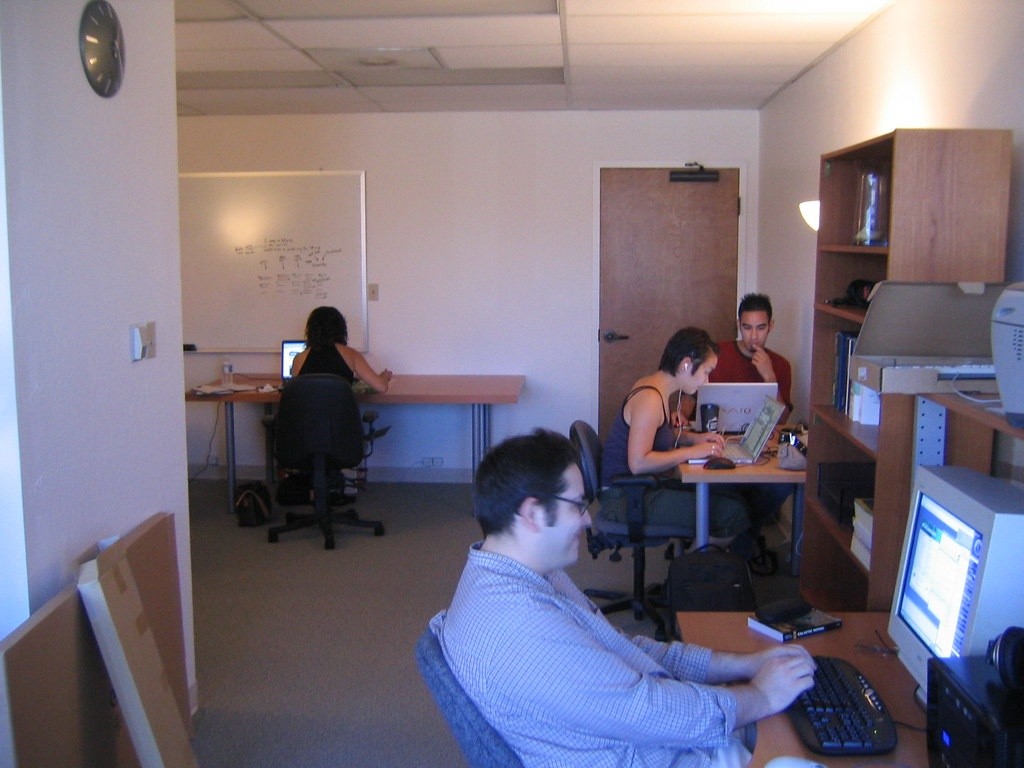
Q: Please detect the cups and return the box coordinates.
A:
[700,404,719,435]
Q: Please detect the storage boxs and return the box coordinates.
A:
[1,510,194,767]
[816,461,875,531]
[850,281,1007,394]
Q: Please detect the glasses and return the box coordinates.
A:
[538,492,591,517]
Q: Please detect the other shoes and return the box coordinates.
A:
[746,549,773,576]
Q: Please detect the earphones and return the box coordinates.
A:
[685,363,688,371]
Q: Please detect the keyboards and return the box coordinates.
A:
[786,656,898,756]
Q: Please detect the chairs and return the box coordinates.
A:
[258,374,384,549]
[569,421,699,641]
[412,622,529,767]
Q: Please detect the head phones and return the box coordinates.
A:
[985,627,1024,696]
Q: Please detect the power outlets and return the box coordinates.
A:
[368,283,379,301]
[134,320,157,360]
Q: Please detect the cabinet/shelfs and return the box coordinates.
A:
[800,127,1011,613]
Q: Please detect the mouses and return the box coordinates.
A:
[764,755,829,768]
[675,417,683,428]
[703,458,735,469]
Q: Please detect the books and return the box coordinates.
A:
[853,174,889,246]
[832,329,881,425]
[748,598,842,644]
[193,382,256,396]
[850,498,875,571]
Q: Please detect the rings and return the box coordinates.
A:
[712,444,715,449]
[711,450,714,455]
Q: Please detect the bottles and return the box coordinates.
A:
[221,355,233,388]
[852,170,889,248]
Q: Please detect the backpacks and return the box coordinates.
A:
[663,541,757,637]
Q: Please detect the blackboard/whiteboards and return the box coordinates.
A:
[178,171,370,352]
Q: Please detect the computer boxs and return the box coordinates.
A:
[925,655,1024,768]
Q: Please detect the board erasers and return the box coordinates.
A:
[183,344,197,352]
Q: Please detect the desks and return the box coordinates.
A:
[674,612,930,767]
[185,373,526,518]
[669,421,806,579]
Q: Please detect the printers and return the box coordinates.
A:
[988,283,1024,429]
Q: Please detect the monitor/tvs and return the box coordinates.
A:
[888,465,1024,703]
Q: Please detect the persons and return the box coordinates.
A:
[670,293,792,424]
[271,307,392,525]
[442,428,818,768]
[599,326,749,552]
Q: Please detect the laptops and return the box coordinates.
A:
[689,383,778,433]
[688,396,786,465]
[278,340,306,391]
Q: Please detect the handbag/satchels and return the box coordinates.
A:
[235,480,276,527]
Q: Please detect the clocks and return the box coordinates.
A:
[79,1,125,96]
